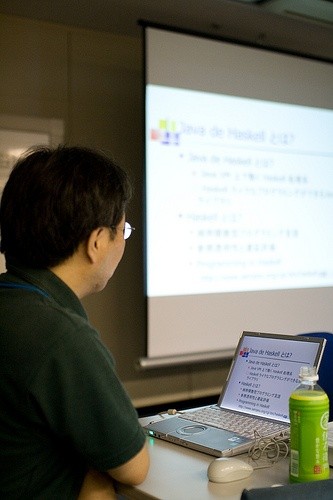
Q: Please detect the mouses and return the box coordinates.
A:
[207,457,253,483]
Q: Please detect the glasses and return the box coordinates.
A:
[111,222,136,239]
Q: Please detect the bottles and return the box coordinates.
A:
[289,365,329,481]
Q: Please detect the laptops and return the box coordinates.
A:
[143,330,327,458]
